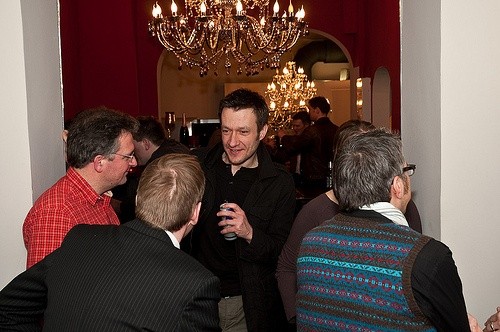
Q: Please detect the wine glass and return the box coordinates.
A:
[165,112,176,140]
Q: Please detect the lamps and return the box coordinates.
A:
[147,0,333,135]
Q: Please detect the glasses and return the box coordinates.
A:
[106,152,135,161]
[399,164,416,176]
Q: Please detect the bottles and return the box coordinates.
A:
[195,118,204,147]
[179,113,190,149]
[327,161,332,188]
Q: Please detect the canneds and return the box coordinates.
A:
[219,202,239,242]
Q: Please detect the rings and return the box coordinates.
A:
[490,322,495,331]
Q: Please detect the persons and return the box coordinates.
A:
[199,87,294,332]
[61,95,341,202]
[0,153,222,332]
[482,306,500,332]
[23,108,138,269]
[294,125,483,332]
[273,119,424,332]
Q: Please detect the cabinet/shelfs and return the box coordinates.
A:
[222,81,350,126]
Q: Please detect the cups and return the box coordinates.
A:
[278,136,283,148]
